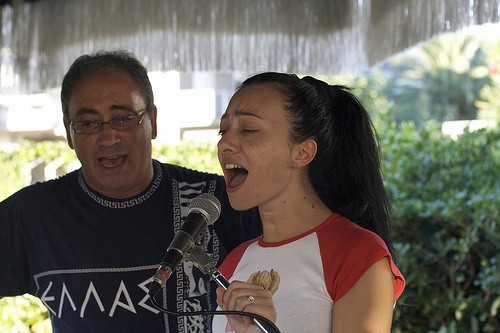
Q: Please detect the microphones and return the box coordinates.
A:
[148,193,221,297]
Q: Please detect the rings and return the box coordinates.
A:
[248,296,255,304]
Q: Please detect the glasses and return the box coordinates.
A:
[68,109,147,135]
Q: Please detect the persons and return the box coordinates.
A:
[212,72,405,333]
[0,54,263,333]
